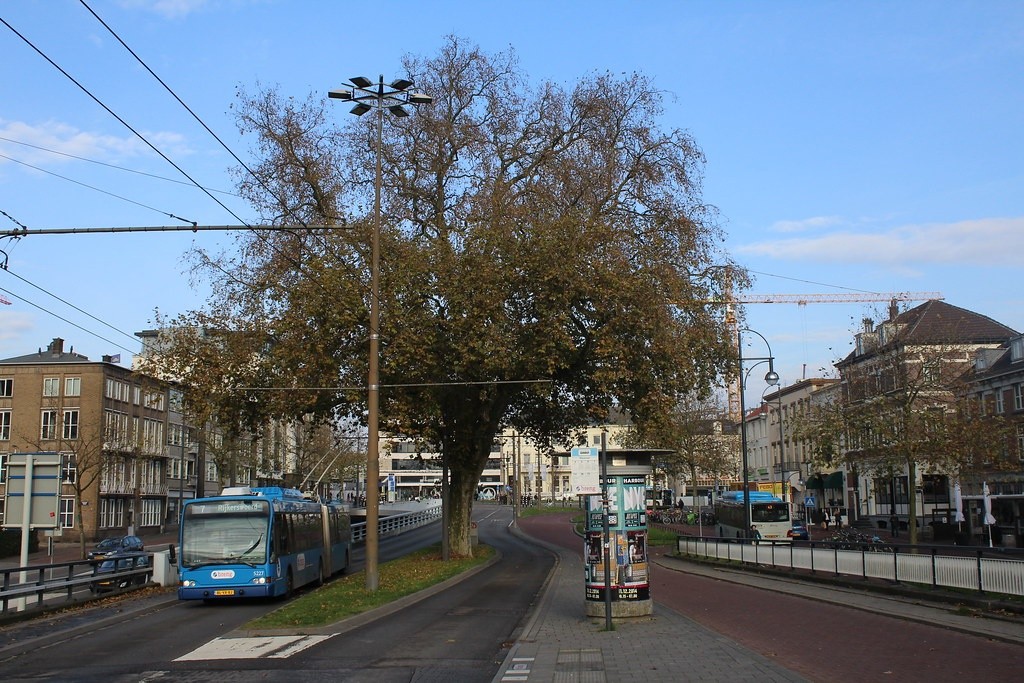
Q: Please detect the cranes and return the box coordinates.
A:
[666,267,945,421]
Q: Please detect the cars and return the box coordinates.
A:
[88,551,153,593]
[792,522,808,540]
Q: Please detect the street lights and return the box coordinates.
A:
[736,328,781,543]
[325,76,439,593]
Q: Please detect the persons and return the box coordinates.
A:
[834,511,842,527]
[889,512,899,537]
[678,499,684,509]
[521,494,537,505]
[547,495,573,507]
[822,511,831,529]
[749,525,761,545]
[347,491,355,504]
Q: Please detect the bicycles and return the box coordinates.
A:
[650,506,715,526]
[821,528,894,553]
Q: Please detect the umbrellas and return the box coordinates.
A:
[955,479,965,531]
[983,482,996,547]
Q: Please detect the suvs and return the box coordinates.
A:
[86,535,145,566]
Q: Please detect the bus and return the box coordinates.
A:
[646,486,675,509]
[169,487,353,602]
[714,490,793,547]
